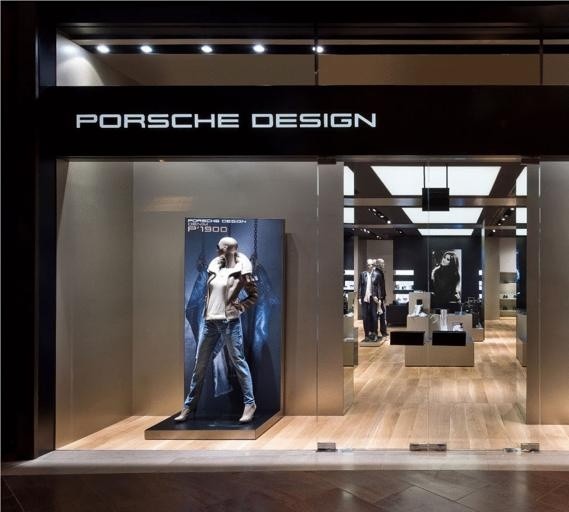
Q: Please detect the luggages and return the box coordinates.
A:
[463,297,479,326]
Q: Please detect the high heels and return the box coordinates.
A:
[174,408,197,422]
[239,403,257,422]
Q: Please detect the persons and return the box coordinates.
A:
[375,258,389,337]
[173,235,260,424]
[357,258,383,342]
[430,250,462,313]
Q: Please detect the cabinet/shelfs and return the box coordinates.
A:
[405,291,485,367]
